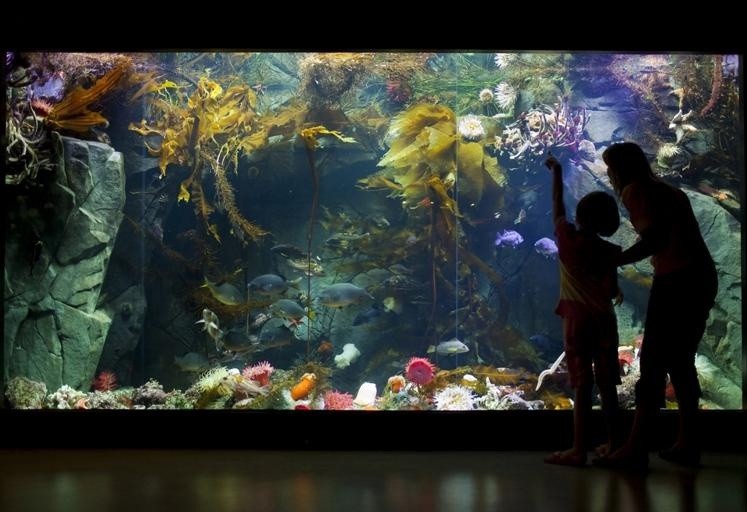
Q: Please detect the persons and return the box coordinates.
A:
[589,143,716,468]
[542,149,622,467]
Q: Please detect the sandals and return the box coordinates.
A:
[544,448,585,466]
[591,450,636,470]
